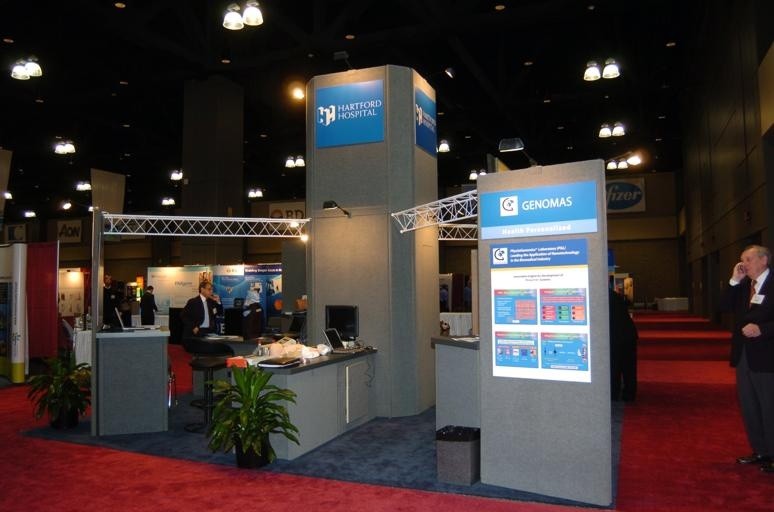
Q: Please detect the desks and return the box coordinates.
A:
[440,313,473,336]
[131,316,169,328]
[72,328,145,369]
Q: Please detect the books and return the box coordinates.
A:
[257,358,300,369]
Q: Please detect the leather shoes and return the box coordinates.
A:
[737,452,770,465]
[759,461,774,472]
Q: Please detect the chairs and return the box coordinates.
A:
[184,335,233,435]
[221,301,306,345]
[62,318,74,364]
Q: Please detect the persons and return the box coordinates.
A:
[609,287,638,403]
[103,273,119,329]
[180,281,224,337]
[139,286,158,326]
[716,244,774,473]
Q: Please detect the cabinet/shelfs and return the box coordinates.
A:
[232,346,376,460]
[96,325,171,436]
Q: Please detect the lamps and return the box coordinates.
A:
[323,200,351,218]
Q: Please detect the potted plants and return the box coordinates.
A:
[23,353,91,433]
[202,360,301,469]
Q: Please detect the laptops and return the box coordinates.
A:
[114,307,145,330]
[321,328,364,353]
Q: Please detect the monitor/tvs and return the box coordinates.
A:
[326,305,358,340]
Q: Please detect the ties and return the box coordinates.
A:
[747,279,756,309]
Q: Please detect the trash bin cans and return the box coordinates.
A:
[435,425,480,487]
